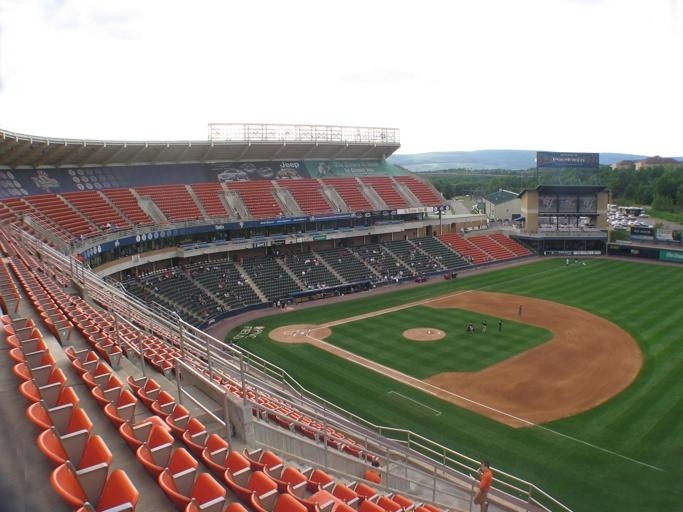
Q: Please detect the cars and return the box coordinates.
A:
[605,204,643,232]
[550,215,589,225]
[216,167,248,182]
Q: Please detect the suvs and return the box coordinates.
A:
[277,168,299,179]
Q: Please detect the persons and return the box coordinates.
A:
[278,274,284,279]
[272,249,285,261]
[145,272,177,292]
[482,318,489,333]
[287,250,343,299]
[518,304,523,318]
[345,241,457,287]
[498,318,504,333]
[198,265,249,307]
[272,300,287,311]
[566,258,586,266]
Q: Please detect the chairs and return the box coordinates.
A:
[289,404,442,512]
[0,278,196,511]
[196,278,373,323]
[447,227,533,270]
[196,361,288,512]
[0,173,446,278]
